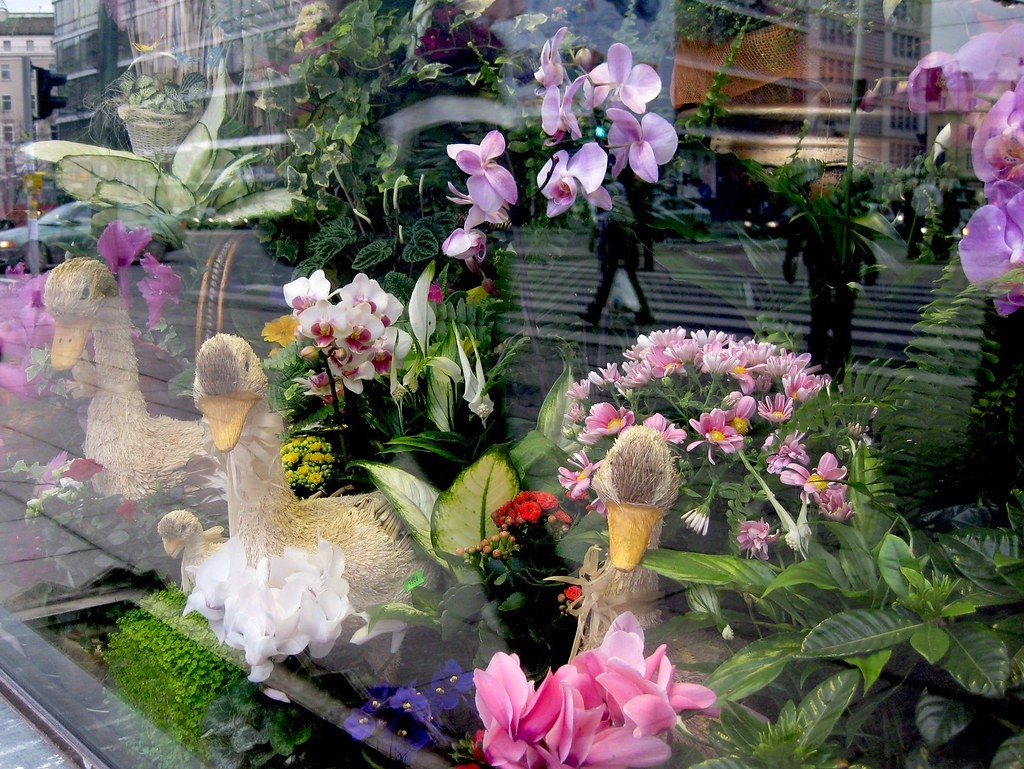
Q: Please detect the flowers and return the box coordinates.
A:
[0,0,1024,769]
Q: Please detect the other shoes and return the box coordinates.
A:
[629,317,656,326]
[828,372,842,384]
[577,313,601,326]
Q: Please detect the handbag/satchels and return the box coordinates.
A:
[605,268,643,313]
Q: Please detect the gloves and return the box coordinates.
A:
[783,267,796,284]
[865,277,877,287]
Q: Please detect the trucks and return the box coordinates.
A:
[0,173,75,229]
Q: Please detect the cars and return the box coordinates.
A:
[591,197,713,242]
[0,200,184,272]
[744,201,793,237]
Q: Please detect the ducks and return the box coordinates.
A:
[192,332,443,686]
[44,256,204,502]
[158,509,229,585]
[577,424,754,769]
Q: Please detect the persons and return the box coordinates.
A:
[899,176,959,265]
[781,174,881,390]
[576,158,665,328]
